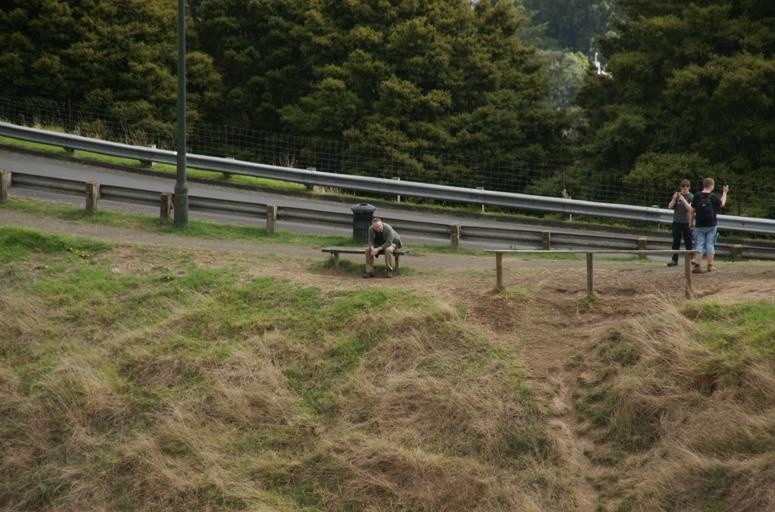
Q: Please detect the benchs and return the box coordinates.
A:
[322,245,409,271]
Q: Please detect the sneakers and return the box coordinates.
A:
[693,269,701,273]
[668,262,677,266]
[708,267,716,272]
[385,269,393,278]
[363,272,374,278]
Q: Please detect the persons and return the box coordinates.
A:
[689,177,730,273]
[362,218,402,277]
[667,179,694,266]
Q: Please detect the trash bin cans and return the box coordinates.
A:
[351,203,376,244]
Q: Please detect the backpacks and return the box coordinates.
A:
[695,192,716,226]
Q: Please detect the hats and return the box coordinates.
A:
[679,179,691,186]
